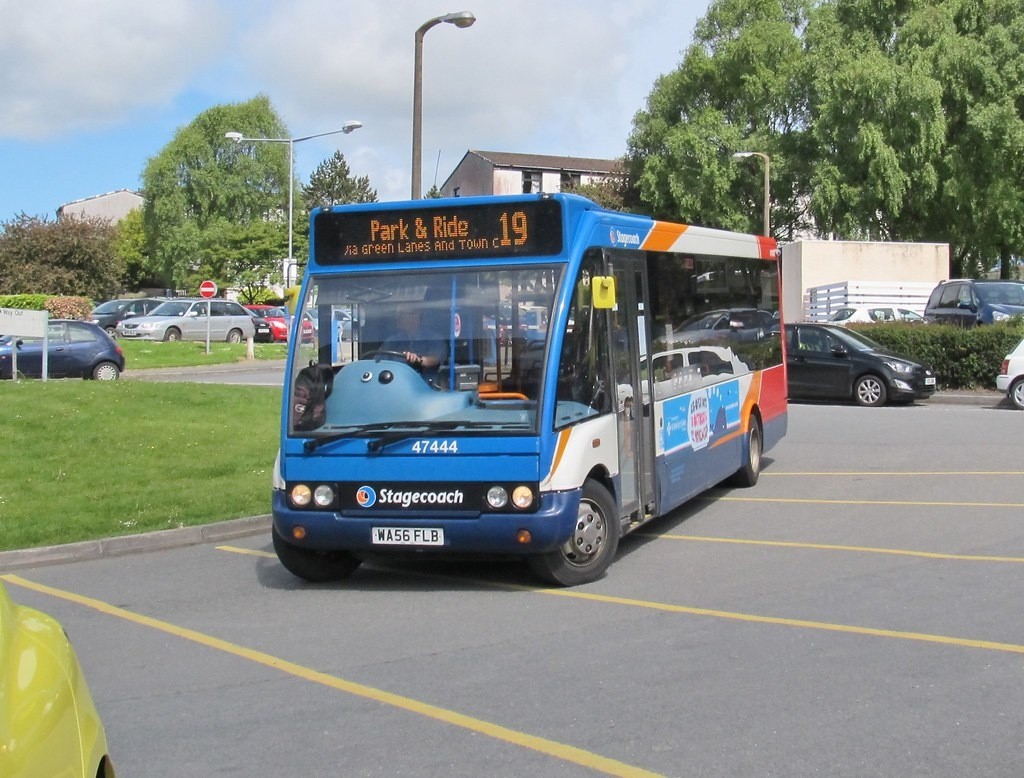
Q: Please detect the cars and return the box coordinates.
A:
[334,309,359,342]
[244,305,292,342]
[0,586,115,778]
[828,304,924,326]
[116,299,255,343]
[278,306,315,343]
[0,319,124,381]
[996,337,1024,410]
[303,309,343,338]
[784,323,935,407]
[245,308,275,342]
[90,299,186,340]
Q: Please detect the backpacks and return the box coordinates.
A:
[293,359,334,431]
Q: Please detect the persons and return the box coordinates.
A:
[377,298,447,377]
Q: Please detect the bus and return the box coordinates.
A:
[271,192,788,587]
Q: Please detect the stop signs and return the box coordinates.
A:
[200,281,215,298]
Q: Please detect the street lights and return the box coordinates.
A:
[733,152,770,237]
[412,11,476,198]
[286,263,306,288]
[225,120,362,260]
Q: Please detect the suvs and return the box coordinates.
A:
[923,279,1024,329]
